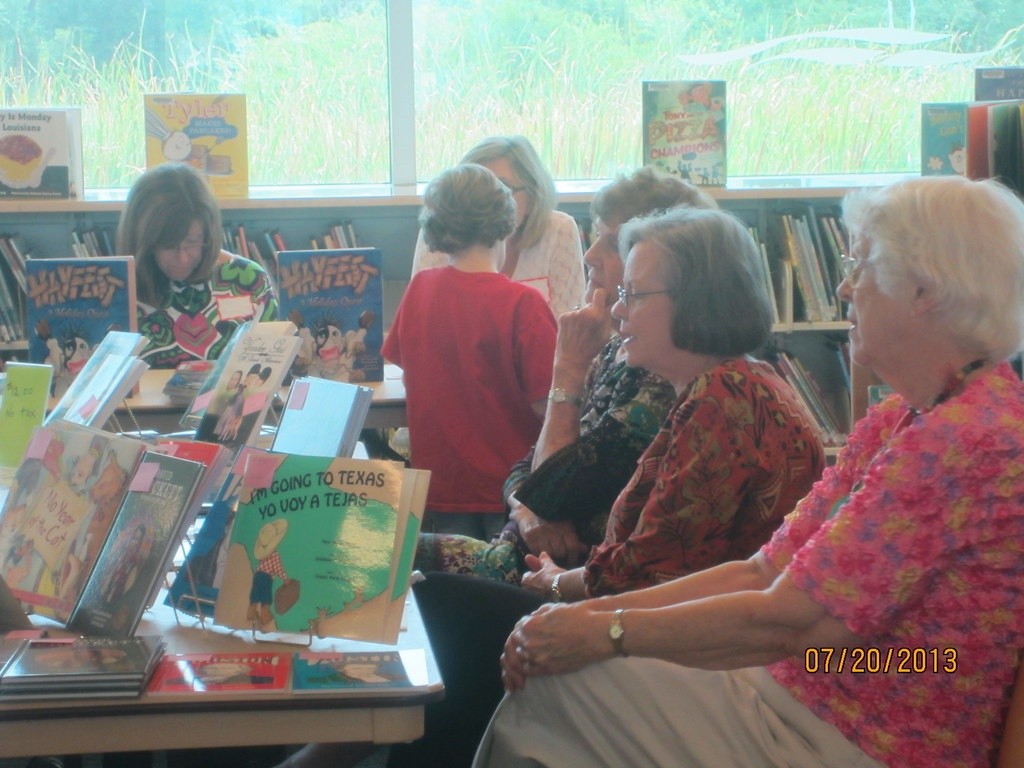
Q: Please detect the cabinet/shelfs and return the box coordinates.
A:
[556,184,852,469]
[0,185,423,352]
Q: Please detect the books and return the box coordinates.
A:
[920,65,1024,201]
[144,93,250,199]
[748,205,856,325]
[775,339,894,450]
[0,106,84,198]
[578,223,588,252]
[642,79,727,187]
[1,222,432,700]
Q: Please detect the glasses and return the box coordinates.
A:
[617,285,671,307]
[506,183,529,195]
[840,254,870,278]
[161,239,211,256]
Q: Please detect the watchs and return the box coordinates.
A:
[551,573,562,601]
[607,610,627,655]
[547,387,585,407]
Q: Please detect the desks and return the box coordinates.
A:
[1,442,446,768]
[46,363,408,434]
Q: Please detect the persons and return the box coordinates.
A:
[375,137,1024,768]
[114,162,278,368]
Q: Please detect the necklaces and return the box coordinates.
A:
[909,359,990,418]
